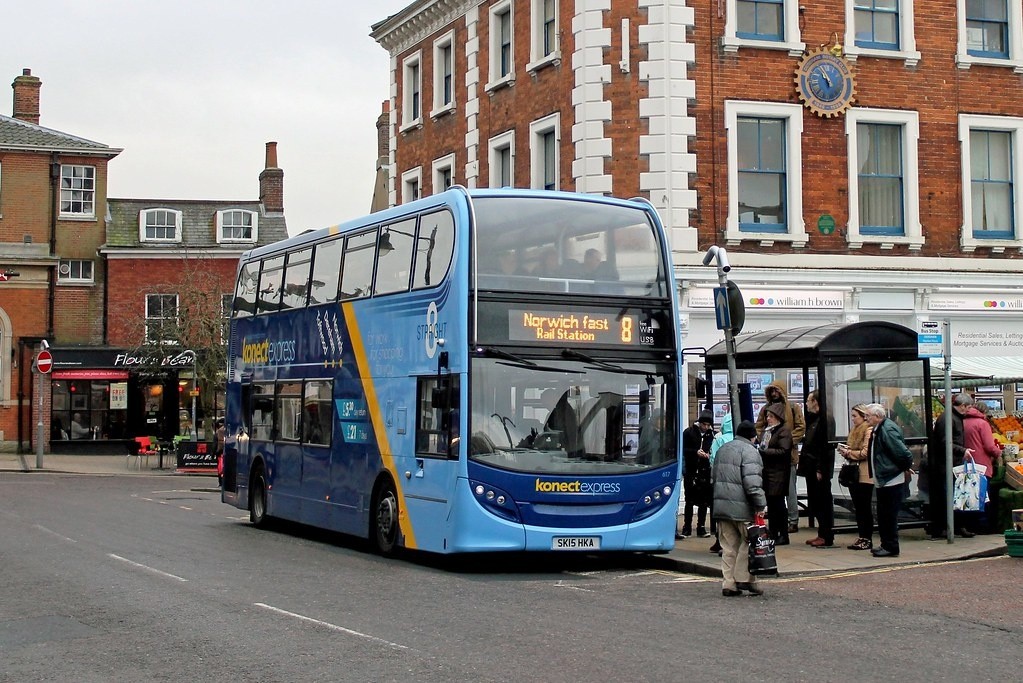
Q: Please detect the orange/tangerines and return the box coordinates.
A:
[1015,465,1023,474]
[991,416,1023,437]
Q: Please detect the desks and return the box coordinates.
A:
[151,441,172,471]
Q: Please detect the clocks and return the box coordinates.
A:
[794,47,858,118]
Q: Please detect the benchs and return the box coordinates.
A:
[796,493,926,522]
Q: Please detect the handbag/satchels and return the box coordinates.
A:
[953,456,991,512]
[838,465,860,487]
[746,515,778,575]
[907,473,919,500]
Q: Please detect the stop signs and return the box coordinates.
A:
[36,350,53,374]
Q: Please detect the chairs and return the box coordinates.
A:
[126,436,190,471]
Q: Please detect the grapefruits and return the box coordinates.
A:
[993,433,1023,458]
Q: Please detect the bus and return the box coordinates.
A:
[216,183,712,559]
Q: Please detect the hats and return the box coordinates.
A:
[698,410,712,423]
[735,420,758,438]
[765,402,786,420]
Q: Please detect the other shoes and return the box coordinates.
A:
[696,529,711,538]
[847,537,864,549]
[954,528,976,538]
[811,538,834,546]
[772,535,790,546]
[722,589,743,597]
[806,537,821,545]
[788,524,799,533]
[675,534,685,540]
[873,549,899,557]
[680,526,692,538]
[736,583,760,595]
[709,540,722,551]
[871,546,884,553]
[853,538,873,549]
[931,532,948,541]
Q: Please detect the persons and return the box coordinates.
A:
[541,388,586,457]
[650,407,666,462]
[799,390,836,547]
[962,403,1003,533]
[930,393,975,540]
[864,404,913,558]
[709,413,734,467]
[571,249,601,290]
[756,380,805,533]
[838,404,875,550]
[759,402,793,546]
[533,246,559,276]
[216,426,225,487]
[712,421,766,595]
[481,247,530,287]
[72,412,89,440]
[682,409,717,538]
[441,409,460,453]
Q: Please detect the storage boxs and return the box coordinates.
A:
[1004,462,1023,491]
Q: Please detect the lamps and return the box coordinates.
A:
[3,266,20,276]
[820,31,846,59]
